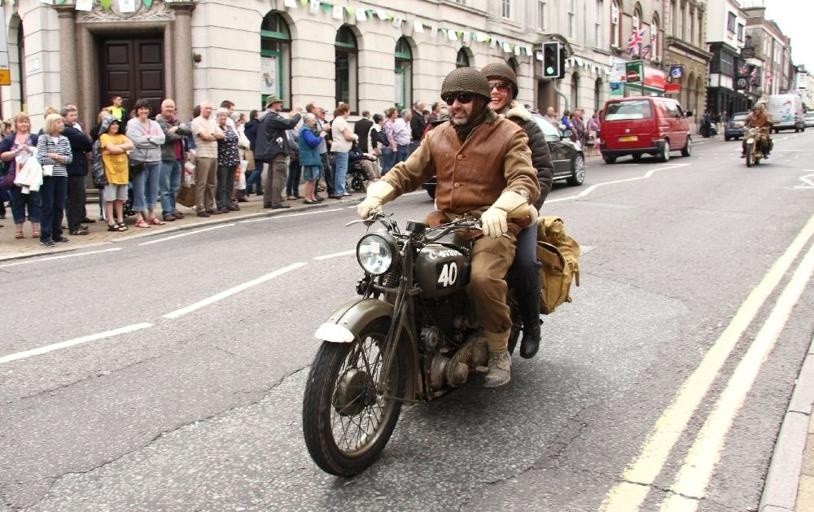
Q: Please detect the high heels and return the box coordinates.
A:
[309,198,320,204]
[303,199,317,204]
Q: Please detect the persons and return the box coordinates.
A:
[479,62,552,358]
[548,107,605,156]
[699,109,727,138]
[740,102,775,155]
[0,96,448,249]
[358,67,535,389]
[742,104,773,159]
[684,109,688,116]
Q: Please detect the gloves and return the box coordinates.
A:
[479,189,528,239]
[356,179,396,224]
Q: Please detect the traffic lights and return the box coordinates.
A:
[542,42,558,77]
[559,48,566,79]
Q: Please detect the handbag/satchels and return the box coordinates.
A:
[128,159,145,175]
[535,213,581,314]
[0,173,14,192]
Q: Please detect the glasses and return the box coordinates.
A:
[488,81,514,92]
[444,92,473,105]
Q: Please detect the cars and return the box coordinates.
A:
[725,112,753,140]
[803,111,814,128]
[421,113,587,196]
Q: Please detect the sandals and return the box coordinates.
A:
[14,230,24,239]
[134,219,152,228]
[148,218,167,225]
[31,229,42,238]
[116,220,130,230]
[107,221,124,232]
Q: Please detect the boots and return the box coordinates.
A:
[519,288,544,361]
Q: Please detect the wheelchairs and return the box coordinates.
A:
[326,144,376,189]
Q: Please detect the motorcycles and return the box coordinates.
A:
[303,204,544,475]
[741,126,769,165]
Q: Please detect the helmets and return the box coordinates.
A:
[752,104,763,111]
[482,62,519,97]
[439,66,492,103]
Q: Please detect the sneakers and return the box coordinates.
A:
[39,236,57,248]
[198,210,211,217]
[207,208,223,215]
[172,211,185,220]
[226,203,242,211]
[70,229,89,235]
[52,233,69,242]
[163,213,177,221]
[218,206,231,213]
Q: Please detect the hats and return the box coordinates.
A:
[264,94,285,109]
[319,107,329,113]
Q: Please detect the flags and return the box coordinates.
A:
[627,28,646,48]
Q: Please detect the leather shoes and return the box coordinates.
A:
[237,198,249,202]
[481,350,514,388]
[263,203,272,208]
[271,202,291,208]
[81,218,97,223]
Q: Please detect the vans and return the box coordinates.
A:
[601,98,692,163]
[768,94,803,132]
